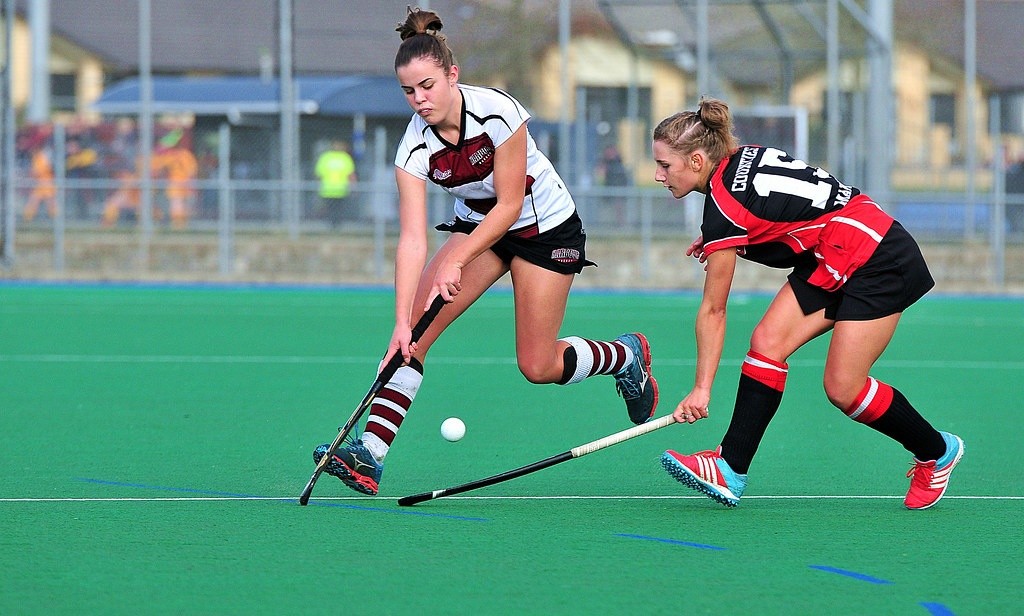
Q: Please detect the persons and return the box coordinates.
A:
[313,7,659,510]
[652,95,966,513]
[314,139,359,227]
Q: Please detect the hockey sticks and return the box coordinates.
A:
[300,290,452,507]
[397,406,709,506]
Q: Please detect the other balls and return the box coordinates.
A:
[440,417,466,442]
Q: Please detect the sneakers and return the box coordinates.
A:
[661,445,747,508]
[314,420,383,495]
[612,333,659,425]
[903,430,968,510]
